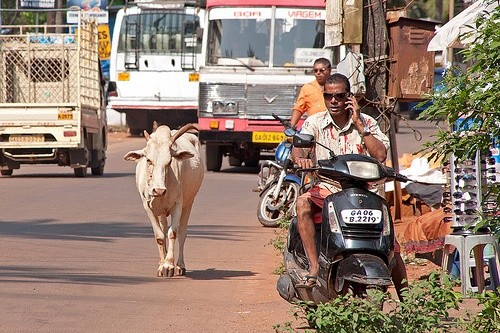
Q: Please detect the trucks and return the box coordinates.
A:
[0,5,118,178]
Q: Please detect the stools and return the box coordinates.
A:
[440,232,500,299]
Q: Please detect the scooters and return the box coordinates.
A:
[252,112,302,228]
[276,133,418,328]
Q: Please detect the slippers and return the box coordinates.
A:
[295,274,317,288]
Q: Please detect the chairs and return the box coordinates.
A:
[121,33,181,52]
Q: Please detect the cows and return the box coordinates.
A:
[122,120,205,278]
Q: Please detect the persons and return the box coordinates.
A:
[292,73,409,323]
[287,58,331,126]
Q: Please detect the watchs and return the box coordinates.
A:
[358,126,370,136]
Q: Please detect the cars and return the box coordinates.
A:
[408,67,465,120]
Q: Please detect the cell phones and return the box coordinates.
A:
[347,93,355,113]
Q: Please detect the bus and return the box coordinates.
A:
[106,0,206,136]
[196,0,347,171]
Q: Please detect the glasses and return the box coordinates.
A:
[314,68,328,73]
[323,92,349,101]
